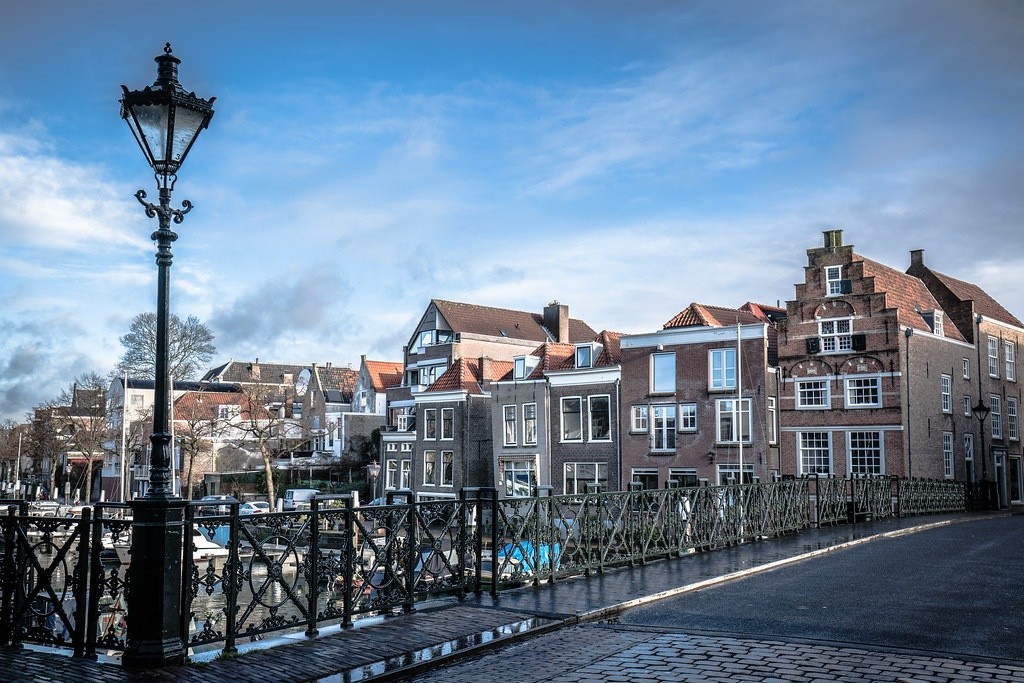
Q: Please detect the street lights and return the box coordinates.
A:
[117,44,216,664]
[972,401,991,480]
[65,464,72,505]
[367,460,380,534]
[465,391,471,485]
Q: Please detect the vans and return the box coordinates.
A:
[284,489,323,510]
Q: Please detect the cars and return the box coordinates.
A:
[197,495,242,515]
[239,501,276,522]
[285,503,325,529]
[362,497,407,521]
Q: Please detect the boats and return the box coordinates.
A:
[299,531,463,593]
[463,539,561,591]
[0,501,241,571]
